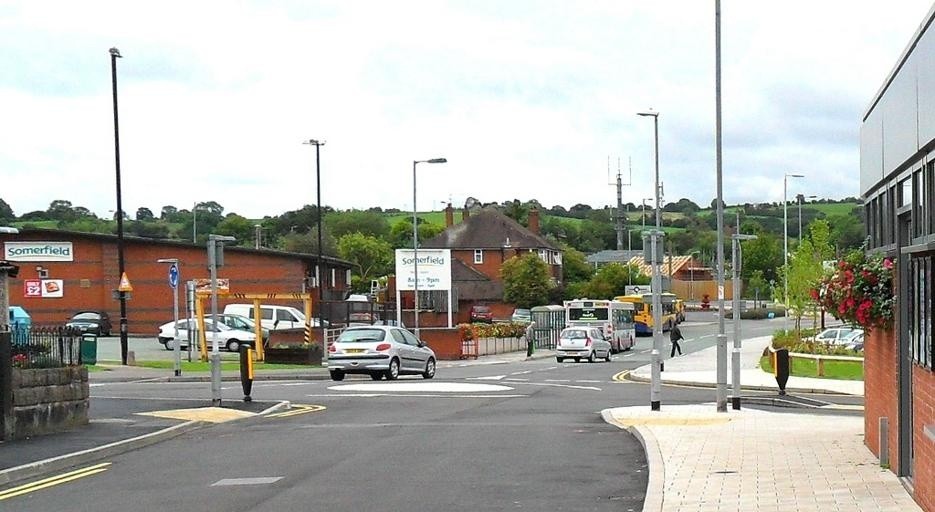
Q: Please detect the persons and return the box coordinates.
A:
[668,321,685,358]
[525,320,536,358]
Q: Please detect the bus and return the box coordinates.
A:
[613,293,686,336]
[565,300,636,353]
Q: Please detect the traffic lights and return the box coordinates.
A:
[371,281,378,299]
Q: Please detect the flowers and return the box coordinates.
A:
[804,235,899,341]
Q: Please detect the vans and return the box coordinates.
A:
[223,302,331,331]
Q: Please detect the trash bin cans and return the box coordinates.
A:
[79,333,97,365]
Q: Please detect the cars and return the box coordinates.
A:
[328,314,437,380]
[63,311,113,337]
[510,308,533,323]
[158,313,272,351]
[470,305,494,324]
[804,302,822,311]
[556,326,613,363]
[801,324,865,354]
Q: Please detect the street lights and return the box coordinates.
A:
[109,47,128,364]
[414,158,447,329]
[636,112,662,228]
[784,174,805,318]
[302,140,328,328]
[798,194,818,246]
